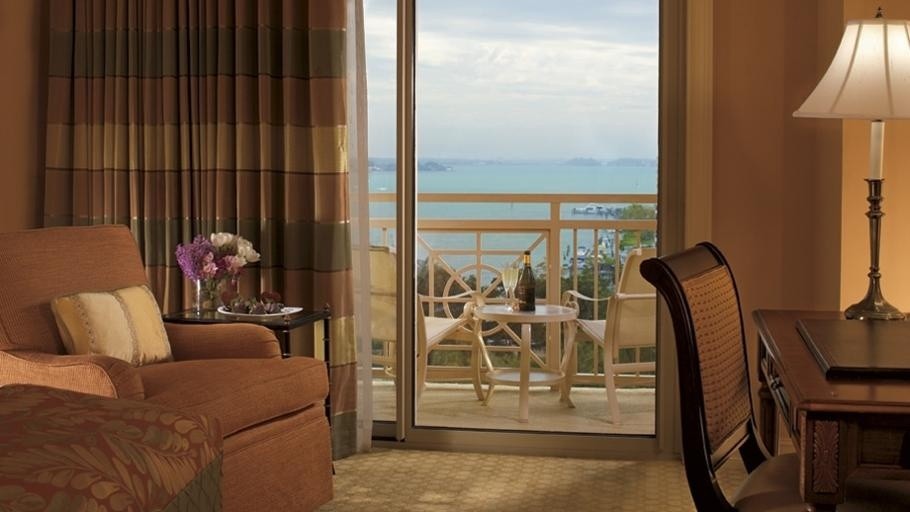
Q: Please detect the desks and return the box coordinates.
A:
[751,308,909,512]
[474,303,577,424]
[163,295,330,424]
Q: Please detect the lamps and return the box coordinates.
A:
[791,6,910,322]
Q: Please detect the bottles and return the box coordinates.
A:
[517,250,536,311]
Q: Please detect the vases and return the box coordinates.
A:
[196,279,225,320]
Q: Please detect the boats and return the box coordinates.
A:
[567,245,616,279]
[574,203,612,215]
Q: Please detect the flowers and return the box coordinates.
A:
[175,232,261,295]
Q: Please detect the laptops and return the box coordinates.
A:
[795,318,910,383]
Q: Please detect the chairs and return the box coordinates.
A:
[639,242,910,511]
[557,247,657,424]
[349,244,486,411]
[1,224,334,510]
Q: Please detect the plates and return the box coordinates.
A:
[216,299,303,323]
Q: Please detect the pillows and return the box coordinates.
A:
[43,284,174,367]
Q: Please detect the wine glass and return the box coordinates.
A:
[501,261,520,311]
